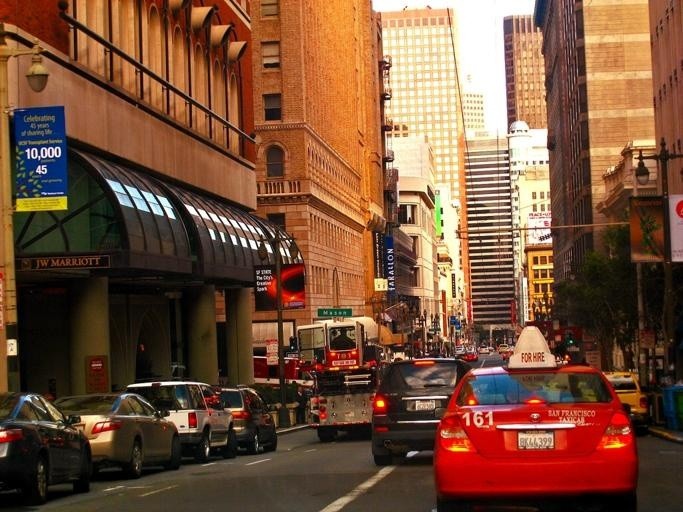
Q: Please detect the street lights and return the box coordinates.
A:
[371,296,441,359]
[1,45,49,394]
[257,224,300,427]
[634,137,683,387]
[532,291,554,342]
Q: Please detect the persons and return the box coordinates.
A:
[625,345,635,372]
[331,327,353,351]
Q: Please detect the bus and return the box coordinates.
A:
[296,316,395,442]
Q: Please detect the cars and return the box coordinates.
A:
[0,380,278,507]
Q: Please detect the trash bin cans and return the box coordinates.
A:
[662,386,683,431]
